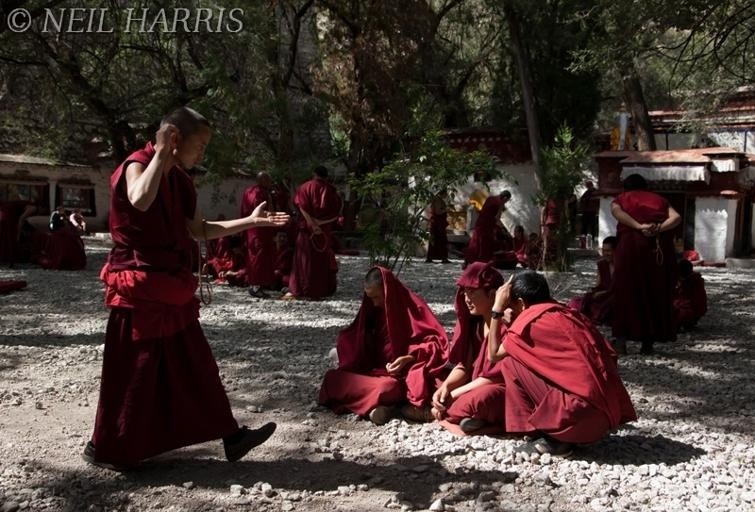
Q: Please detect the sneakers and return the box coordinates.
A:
[223,422,278,464]
[82,443,138,471]
[424,260,434,264]
[284,292,293,298]
[369,405,392,426]
[460,417,484,433]
[609,341,628,355]
[529,438,573,456]
[442,260,451,264]
[249,288,271,298]
[640,343,653,351]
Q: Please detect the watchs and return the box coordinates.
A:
[491,311,505,319]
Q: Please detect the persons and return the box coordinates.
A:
[425,188,451,264]
[0,198,40,267]
[536,194,566,273]
[318,265,450,425]
[400,261,509,435]
[50,219,87,270]
[610,173,682,354]
[82,105,291,470]
[461,190,511,270]
[49,205,68,231]
[205,214,235,284]
[579,181,599,239]
[486,272,638,444]
[280,164,345,300]
[239,171,283,299]
[509,225,529,271]
[69,209,86,234]
[671,251,708,331]
[566,236,617,328]
